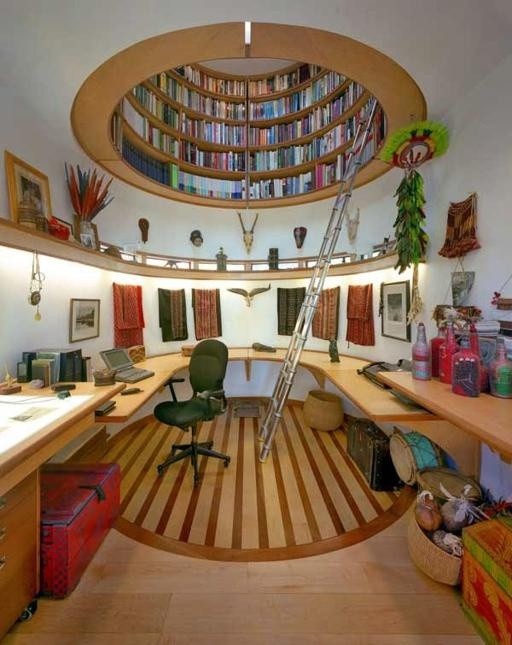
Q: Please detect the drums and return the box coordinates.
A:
[389,431,442,485]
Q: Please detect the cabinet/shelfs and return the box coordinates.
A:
[248,63,388,208]
[112,63,249,209]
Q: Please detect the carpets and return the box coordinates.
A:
[91,397,420,564]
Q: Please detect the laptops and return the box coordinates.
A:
[99,348,155,384]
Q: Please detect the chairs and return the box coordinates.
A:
[153,341,230,483]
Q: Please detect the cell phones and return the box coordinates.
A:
[121,388,144,395]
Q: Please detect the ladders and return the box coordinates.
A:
[258,98,377,464]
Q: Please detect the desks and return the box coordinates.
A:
[132,347,246,402]
[248,346,369,421]
[83,369,170,426]
[371,371,512,463]
[328,371,479,508]
[1,376,126,616]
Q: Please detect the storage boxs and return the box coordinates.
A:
[462,518,512,645]
[36,461,121,599]
[342,419,404,492]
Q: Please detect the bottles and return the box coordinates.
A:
[440,315,459,384]
[431,321,447,377]
[216,247,228,270]
[469,325,488,392]
[269,248,278,271]
[412,322,432,381]
[451,334,481,397]
[488,339,512,399]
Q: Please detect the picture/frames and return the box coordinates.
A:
[5,148,52,234]
[381,280,411,342]
[71,300,102,342]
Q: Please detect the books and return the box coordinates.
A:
[110,61,389,201]
[16,346,93,388]
[95,400,117,417]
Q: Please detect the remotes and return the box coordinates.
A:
[51,384,76,391]
